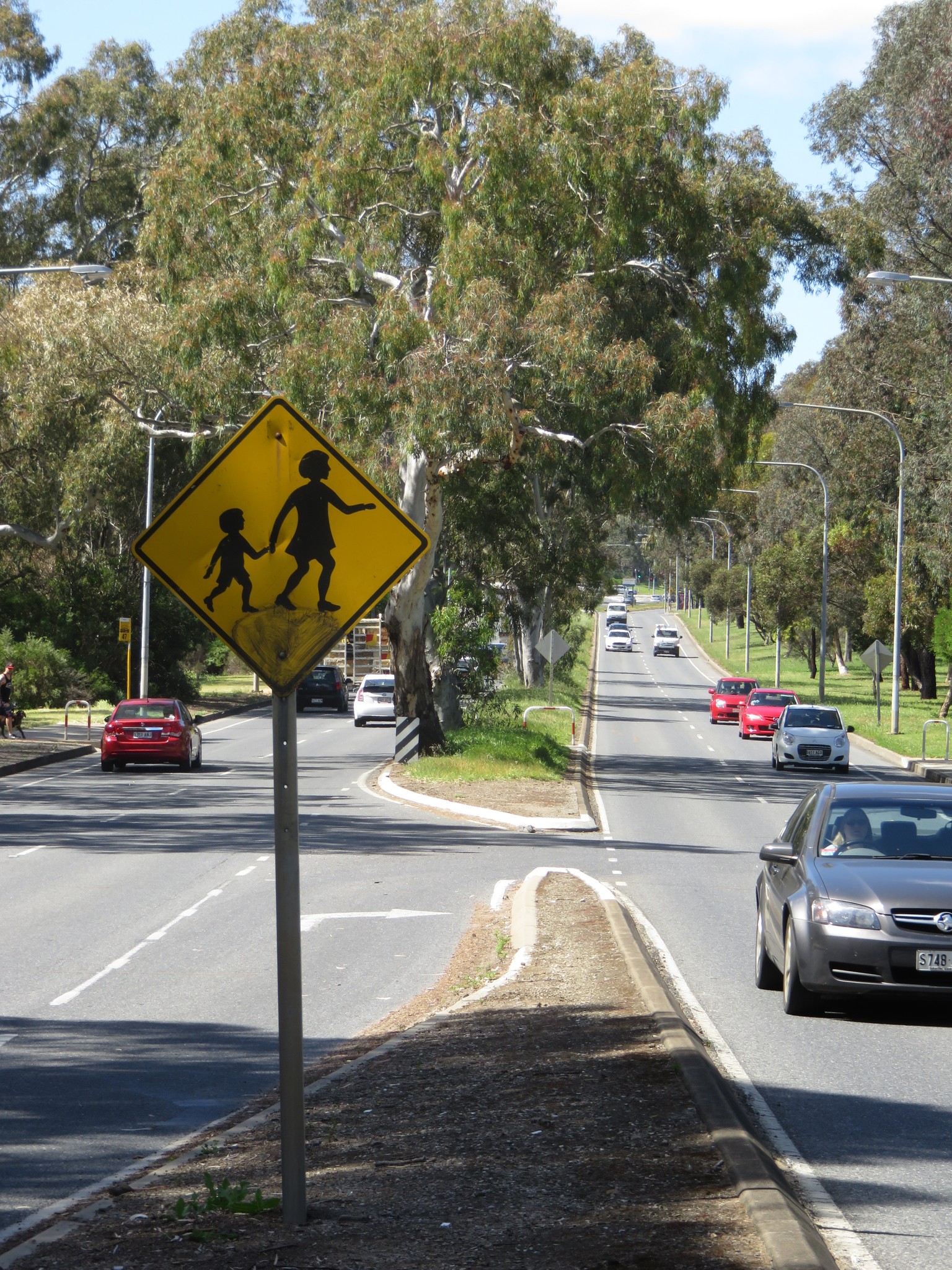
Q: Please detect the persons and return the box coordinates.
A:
[721,687,734,695]
[0,662,22,738]
[670,631,676,637]
[780,696,791,707]
[340,637,353,659]
[658,632,664,637]
[822,806,872,857]
[751,692,767,705]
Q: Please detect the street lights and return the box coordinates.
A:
[773,400,907,736]
[634,515,686,615]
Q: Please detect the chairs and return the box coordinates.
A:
[162,708,172,718]
[324,673,329,681]
[123,710,136,718]
[831,815,874,842]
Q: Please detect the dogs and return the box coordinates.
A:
[0,711,26,739]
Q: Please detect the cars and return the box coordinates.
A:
[708,677,760,724]
[738,688,801,739]
[755,781,952,1014]
[768,704,855,774]
[603,629,634,653]
[101,697,205,772]
[621,593,637,606]
[605,623,632,634]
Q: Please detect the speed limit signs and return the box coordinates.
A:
[119,617,131,641]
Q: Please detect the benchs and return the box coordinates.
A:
[825,821,952,857]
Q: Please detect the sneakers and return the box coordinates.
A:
[9,734,20,740]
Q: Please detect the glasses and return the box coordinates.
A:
[843,820,866,826]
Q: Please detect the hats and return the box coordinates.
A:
[6,662,14,668]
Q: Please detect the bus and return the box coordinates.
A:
[324,613,397,693]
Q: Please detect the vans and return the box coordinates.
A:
[604,602,629,627]
[651,623,682,658]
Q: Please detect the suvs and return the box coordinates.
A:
[353,672,397,728]
[612,585,637,596]
[487,643,510,663]
[296,664,353,713]
[454,654,485,699]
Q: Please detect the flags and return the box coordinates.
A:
[366,634,377,646]
